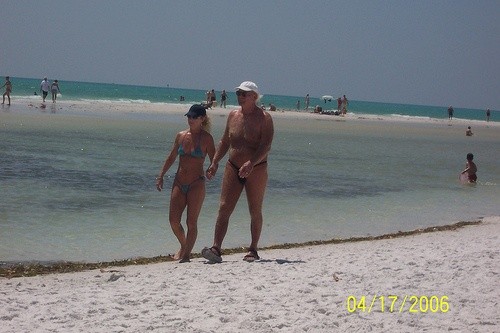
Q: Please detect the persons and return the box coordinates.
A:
[461,153,478,182]
[487,109,490,121]
[50,80,62,103]
[448,106,454,119]
[305,93,310,112]
[466,126,472,136]
[40,78,50,102]
[0,75,12,105]
[202,81,274,263]
[268,103,276,111]
[297,100,301,112]
[220,89,230,108]
[157,104,218,263]
[208,89,216,109]
[261,103,266,110]
[337,95,348,112]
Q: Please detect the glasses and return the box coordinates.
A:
[188,115,198,119]
[236,92,247,96]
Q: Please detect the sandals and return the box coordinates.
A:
[201,247,222,262]
[243,249,259,262]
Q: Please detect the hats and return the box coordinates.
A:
[236,81,258,93]
[184,104,206,116]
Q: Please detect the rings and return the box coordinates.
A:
[246,172,249,174]
